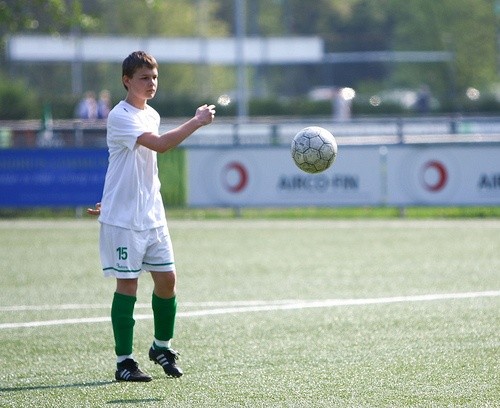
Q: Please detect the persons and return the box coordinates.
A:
[88,52,215,382]
[331,84,353,119]
[409,86,433,114]
[75,88,112,123]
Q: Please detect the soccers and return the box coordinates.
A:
[291,126,338,174]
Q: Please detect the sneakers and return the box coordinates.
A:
[149,341,183,378]
[115,358,152,381]
